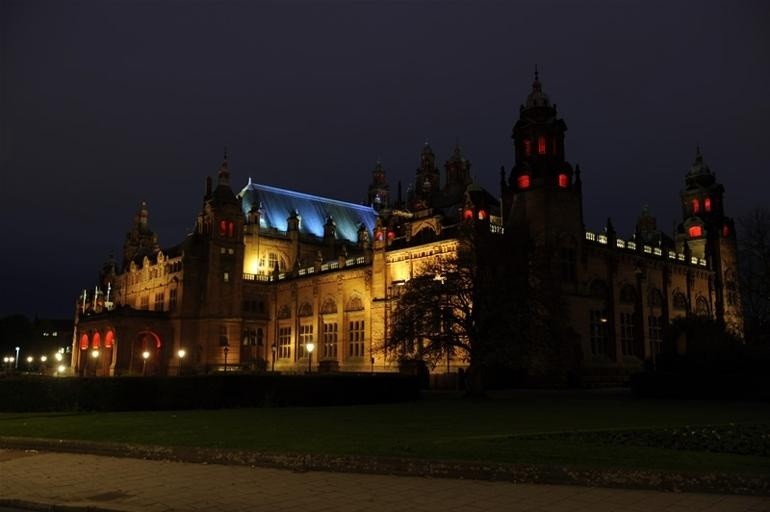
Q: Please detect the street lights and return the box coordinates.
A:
[305,342,314,372]
[223,346,229,371]
[141,351,150,376]
[178,350,185,372]
[270,342,277,372]
